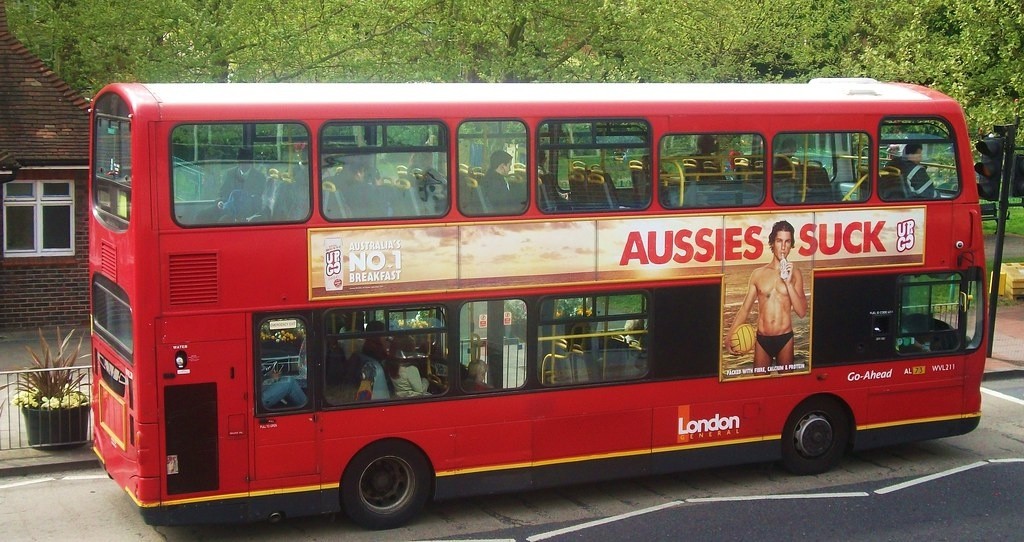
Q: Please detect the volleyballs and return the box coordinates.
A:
[730,324,755,353]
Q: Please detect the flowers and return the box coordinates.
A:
[260,318,306,343]
[0,325,92,417]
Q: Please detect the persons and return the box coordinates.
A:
[885,143,941,200]
[688,135,745,182]
[901,329,932,354]
[726,220,808,376]
[621,318,647,351]
[321,160,391,221]
[539,149,572,209]
[478,150,513,209]
[774,138,809,190]
[196,145,309,222]
[262,321,495,411]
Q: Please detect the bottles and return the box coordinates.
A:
[779,252,789,279]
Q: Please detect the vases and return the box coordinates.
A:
[260,341,299,357]
[20,404,90,450]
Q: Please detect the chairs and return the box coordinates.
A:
[556,333,649,379]
[245,158,912,222]
[347,335,392,407]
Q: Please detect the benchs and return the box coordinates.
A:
[980,203,1010,235]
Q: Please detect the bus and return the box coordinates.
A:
[88,76,991,534]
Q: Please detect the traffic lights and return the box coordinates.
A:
[974,135,1007,203]
